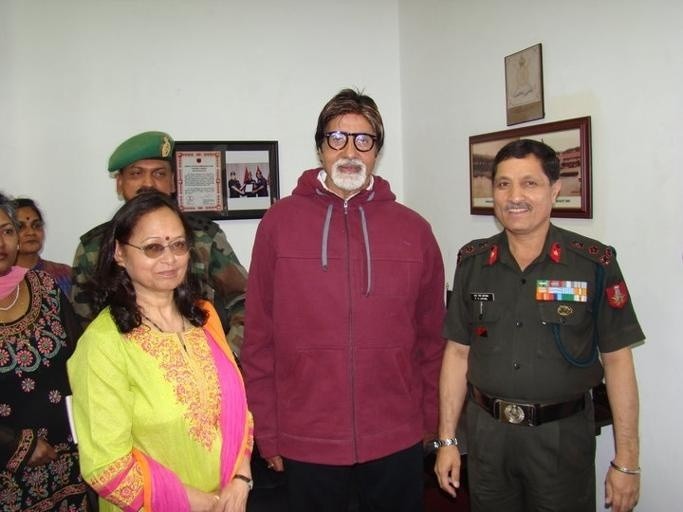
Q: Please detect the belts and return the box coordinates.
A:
[468,384,585,428]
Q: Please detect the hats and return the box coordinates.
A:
[108,132,175,172]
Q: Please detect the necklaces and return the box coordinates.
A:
[138,311,186,351]
[0,282,20,311]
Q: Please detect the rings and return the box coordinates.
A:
[267,463,273,469]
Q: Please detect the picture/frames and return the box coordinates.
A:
[504,43,544,127]
[469,116,592,218]
[172,142,279,220]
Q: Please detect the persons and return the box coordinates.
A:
[9,198,73,304]
[227,165,269,198]
[241,87,448,512]
[0,192,89,512]
[67,188,255,511]
[70,131,248,370]
[433,139,646,512]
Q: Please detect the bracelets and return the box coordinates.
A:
[433,438,458,449]
[232,474,254,490]
[214,495,220,501]
[610,461,641,475]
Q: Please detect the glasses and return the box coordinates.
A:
[123,240,191,259]
[323,130,379,152]
